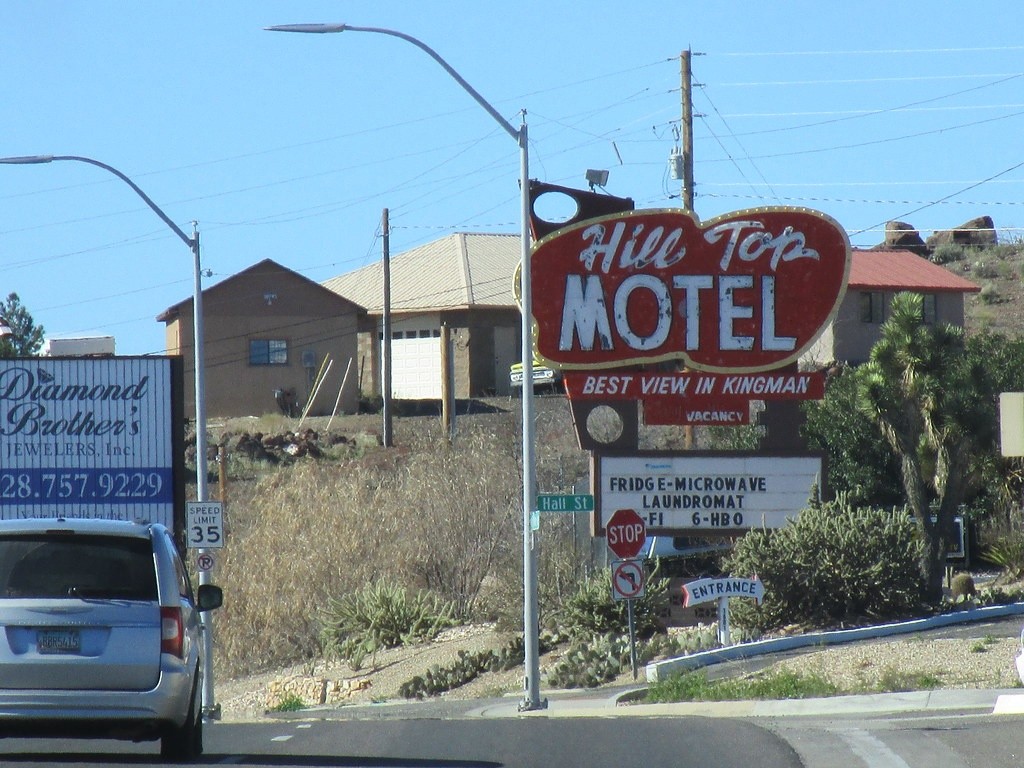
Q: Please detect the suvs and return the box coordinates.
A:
[0,516,219,763]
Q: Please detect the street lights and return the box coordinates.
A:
[263,20,551,714]
[0,150,223,728]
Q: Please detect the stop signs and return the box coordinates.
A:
[607,510,647,559]
